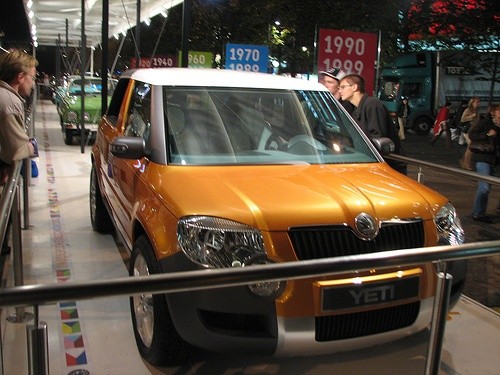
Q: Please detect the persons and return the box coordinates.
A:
[431,103,452,147]
[460,98,478,172]
[337,75,407,176]
[396,98,411,141]
[44,74,52,98]
[0,49,39,255]
[322,68,356,148]
[471,104,500,220]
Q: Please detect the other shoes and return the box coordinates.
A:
[472,214,492,224]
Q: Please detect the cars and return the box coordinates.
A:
[57,77,122,146]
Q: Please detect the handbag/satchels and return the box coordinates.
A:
[457,120,471,134]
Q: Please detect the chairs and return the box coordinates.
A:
[168,105,198,154]
[228,107,268,153]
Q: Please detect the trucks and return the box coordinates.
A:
[377,47,500,136]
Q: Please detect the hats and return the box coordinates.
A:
[319,67,346,80]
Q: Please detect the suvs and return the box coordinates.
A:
[89,67,468,366]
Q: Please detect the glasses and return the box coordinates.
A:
[338,84,353,90]
